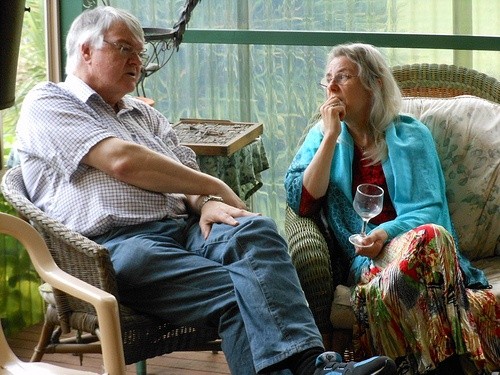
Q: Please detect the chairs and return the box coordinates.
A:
[285,64,500,362]
[0,163,225,375]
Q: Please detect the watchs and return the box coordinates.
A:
[203,195,223,206]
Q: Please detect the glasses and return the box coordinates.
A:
[320,72,360,89]
[102,39,148,61]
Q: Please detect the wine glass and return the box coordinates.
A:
[348,184,384,247]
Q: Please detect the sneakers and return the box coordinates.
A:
[272,352,396,375]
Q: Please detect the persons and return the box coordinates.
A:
[16,6,392,375]
[284,42,500,375]
